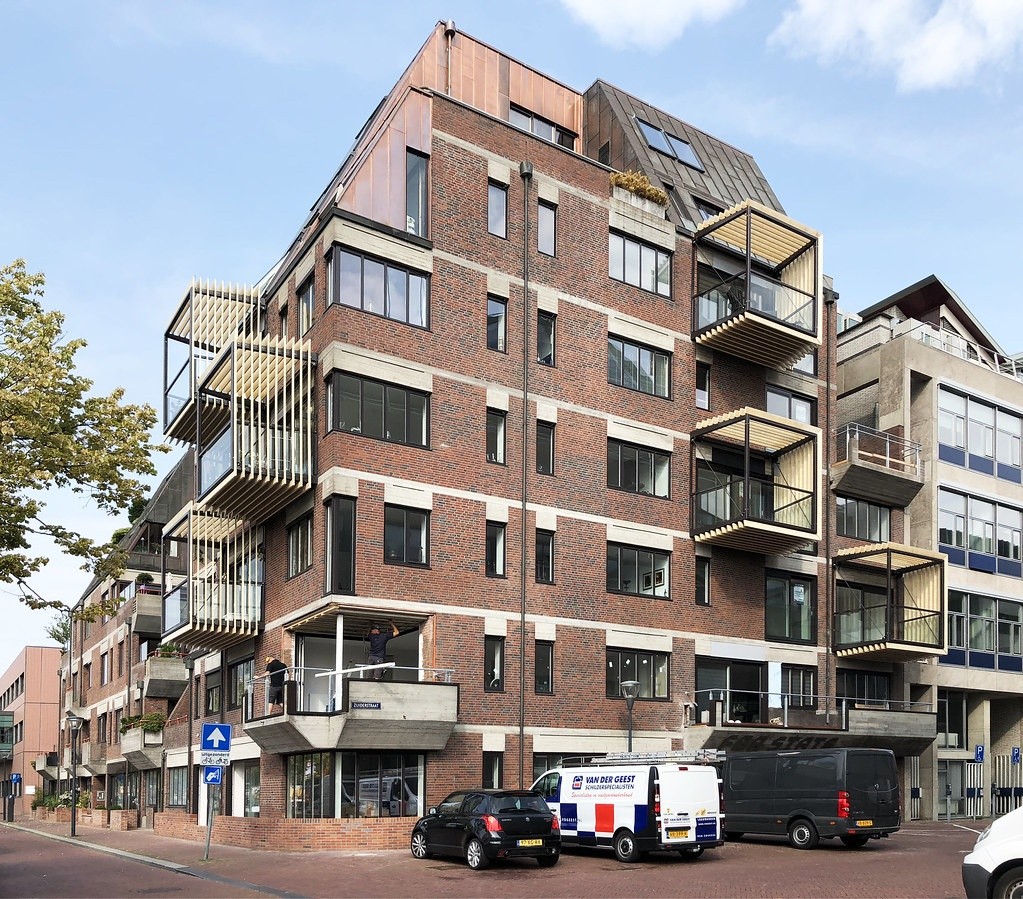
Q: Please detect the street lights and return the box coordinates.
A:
[0,750,11,821]
[619,680,641,752]
[66,716,84,836]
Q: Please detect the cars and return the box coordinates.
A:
[961,806,1023,899]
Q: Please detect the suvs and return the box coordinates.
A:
[410,788,562,871]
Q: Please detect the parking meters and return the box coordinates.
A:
[945,784,952,823]
[992,783,997,818]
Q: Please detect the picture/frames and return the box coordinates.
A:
[643,569,664,591]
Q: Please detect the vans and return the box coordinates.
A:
[691,748,901,850]
[516,761,726,864]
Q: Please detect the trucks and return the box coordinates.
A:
[359,776,417,816]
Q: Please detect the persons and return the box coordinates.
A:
[368,619,399,679]
[252,657,289,715]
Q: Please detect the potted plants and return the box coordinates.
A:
[119,713,163,744]
[91,805,109,828]
[610,169,670,219]
[110,805,128,830]
[28,786,90,821]
[136,572,154,590]
